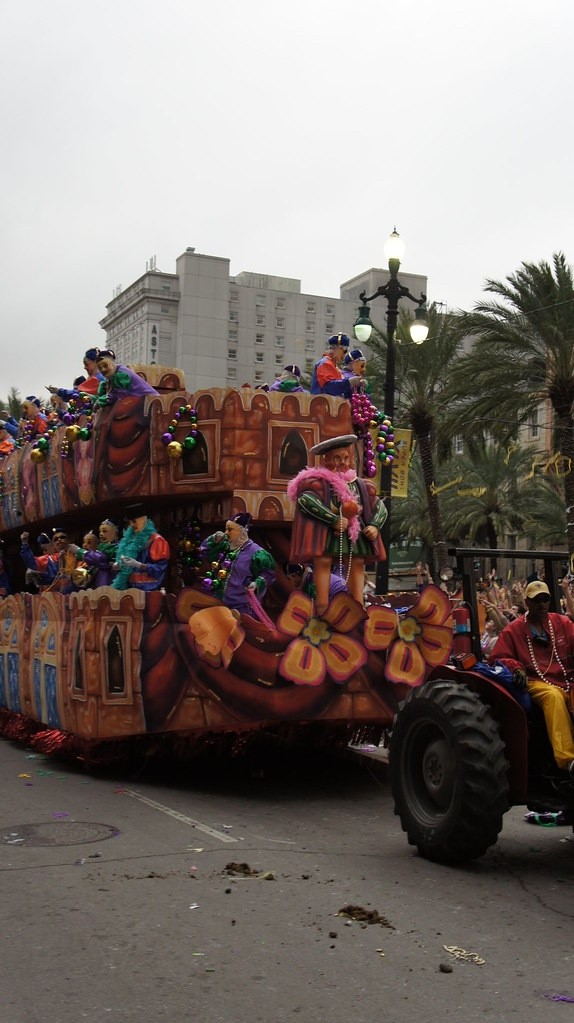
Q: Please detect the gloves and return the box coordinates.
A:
[67,545,81,553]
[213,531,225,543]
[122,557,141,568]
[245,581,256,593]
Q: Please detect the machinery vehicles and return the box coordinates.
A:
[0,367,574,869]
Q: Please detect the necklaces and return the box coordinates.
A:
[340,503,353,585]
[200,546,241,587]
[352,383,394,476]
[0,396,93,515]
[523,611,570,692]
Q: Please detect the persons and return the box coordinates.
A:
[363,572,392,608]
[242,334,368,400]
[0,348,160,456]
[289,563,349,601]
[197,511,278,620]
[486,581,574,777]
[21,525,77,595]
[110,501,171,591]
[415,559,574,656]
[67,516,120,592]
[286,434,389,617]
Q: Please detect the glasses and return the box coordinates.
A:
[341,349,347,354]
[531,595,549,604]
[360,363,366,367]
[53,535,66,542]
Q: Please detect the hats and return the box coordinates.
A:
[124,503,149,520]
[98,350,115,359]
[522,579,552,599]
[74,376,87,385]
[328,335,350,348]
[230,512,253,528]
[82,529,102,536]
[45,527,68,537]
[37,532,53,543]
[86,349,102,363]
[27,396,42,410]
[344,351,365,363]
[104,517,122,530]
[284,365,300,377]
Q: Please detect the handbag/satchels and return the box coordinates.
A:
[525,811,564,827]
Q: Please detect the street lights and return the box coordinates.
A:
[353,226,430,595]
